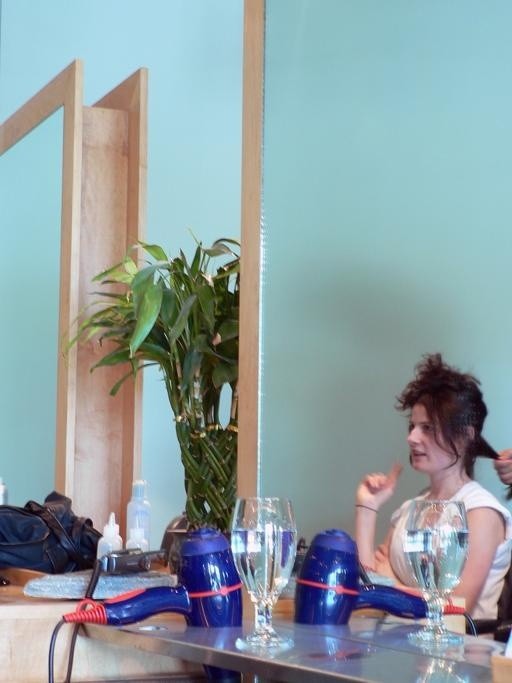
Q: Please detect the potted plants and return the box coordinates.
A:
[58,228,240,531]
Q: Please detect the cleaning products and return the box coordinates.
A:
[127,517,147,549]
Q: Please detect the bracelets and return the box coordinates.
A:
[356,504,379,513]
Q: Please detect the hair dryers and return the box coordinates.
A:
[293,531,468,626]
[62,527,243,628]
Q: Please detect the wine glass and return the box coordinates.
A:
[231,498,299,659]
[403,501,469,648]
[406,640,471,681]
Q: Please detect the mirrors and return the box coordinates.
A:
[0,58,84,508]
[240,0,511,641]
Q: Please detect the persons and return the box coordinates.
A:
[354,350,510,640]
[495,448,512,487]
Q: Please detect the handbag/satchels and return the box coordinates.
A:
[0,490,104,575]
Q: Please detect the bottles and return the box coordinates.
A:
[126,482,152,559]
